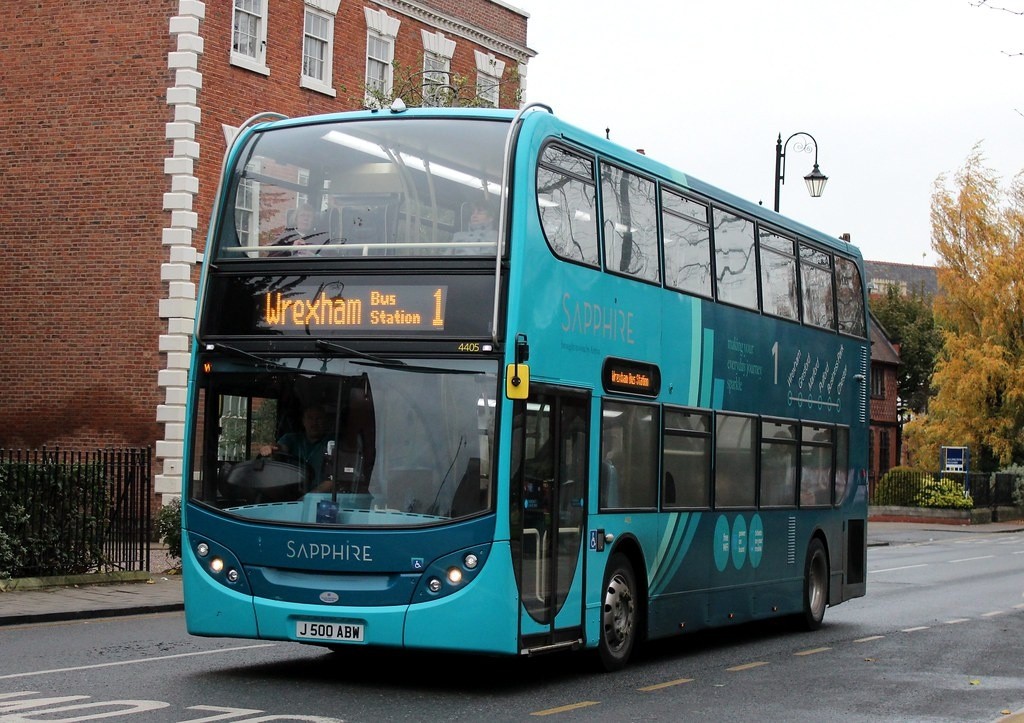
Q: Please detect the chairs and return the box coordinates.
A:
[460,199,499,232]
[286,207,339,244]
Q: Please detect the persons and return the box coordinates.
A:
[256,396,848,597]
[254,193,864,337]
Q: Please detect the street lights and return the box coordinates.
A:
[774,132,828,214]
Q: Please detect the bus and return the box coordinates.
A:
[179,95,871,666]
